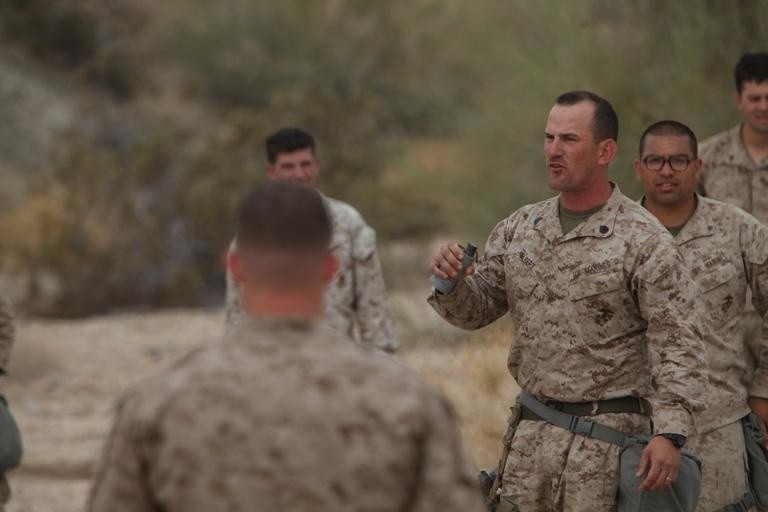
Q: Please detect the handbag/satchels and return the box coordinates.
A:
[618,434,701,512]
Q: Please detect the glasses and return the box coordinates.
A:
[640,154,698,171]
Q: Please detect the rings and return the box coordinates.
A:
[666,474,674,483]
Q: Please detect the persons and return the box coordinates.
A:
[428,90,709,510]
[0,295,28,512]
[628,120,767,510]
[693,50,766,223]
[79,179,489,511]
[225,126,400,357]
